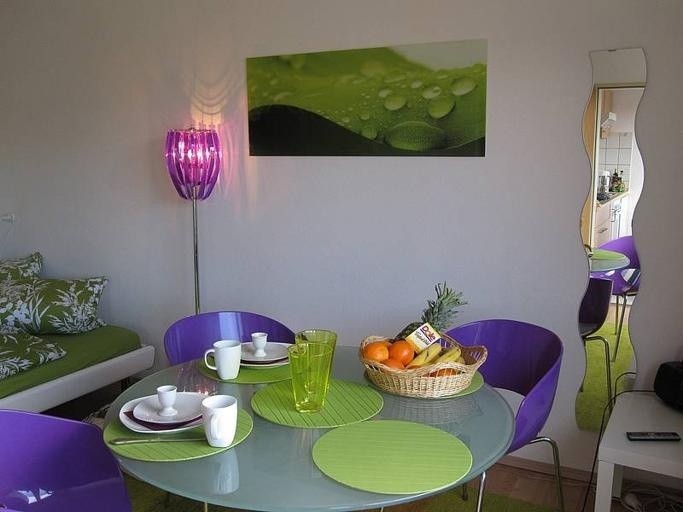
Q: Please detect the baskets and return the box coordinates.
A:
[358,328,488,399]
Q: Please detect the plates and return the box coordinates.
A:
[238,341,293,367]
[135,392,205,426]
[119,393,209,436]
[240,343,289,363]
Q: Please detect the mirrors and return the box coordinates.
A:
[576,47,644,432]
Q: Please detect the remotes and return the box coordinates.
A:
[626,430,681,442]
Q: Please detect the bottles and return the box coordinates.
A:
[612,167,626,192]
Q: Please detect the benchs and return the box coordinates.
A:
[0,252,154,414]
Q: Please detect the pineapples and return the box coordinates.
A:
[393,281,468,339]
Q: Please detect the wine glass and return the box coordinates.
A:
[251,331,269,357]
[155,384,180,418]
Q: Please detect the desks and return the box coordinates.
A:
[594,389,683,512]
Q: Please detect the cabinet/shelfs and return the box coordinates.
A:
[594,194,628,246]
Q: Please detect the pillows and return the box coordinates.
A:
[0,252,108,381]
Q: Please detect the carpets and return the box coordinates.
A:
[575,323,633,429]
[121,472,563,511]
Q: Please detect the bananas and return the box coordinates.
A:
[408,343,467,367]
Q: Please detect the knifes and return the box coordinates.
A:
[110,437,206,446]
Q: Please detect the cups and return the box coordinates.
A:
[206,449,240,495]
[293,329,338,395]
[288,344,332,414]
[205,339,241,379]
[201,394,238,447]
[216,382,244,411]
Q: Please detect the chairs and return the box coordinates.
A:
[578,277,612,415]
[590,237,640,363]
[164,312,296,366]
[0,410,132,512]
[443,318,564,512]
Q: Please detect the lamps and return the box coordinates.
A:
[166,128,223,315]
